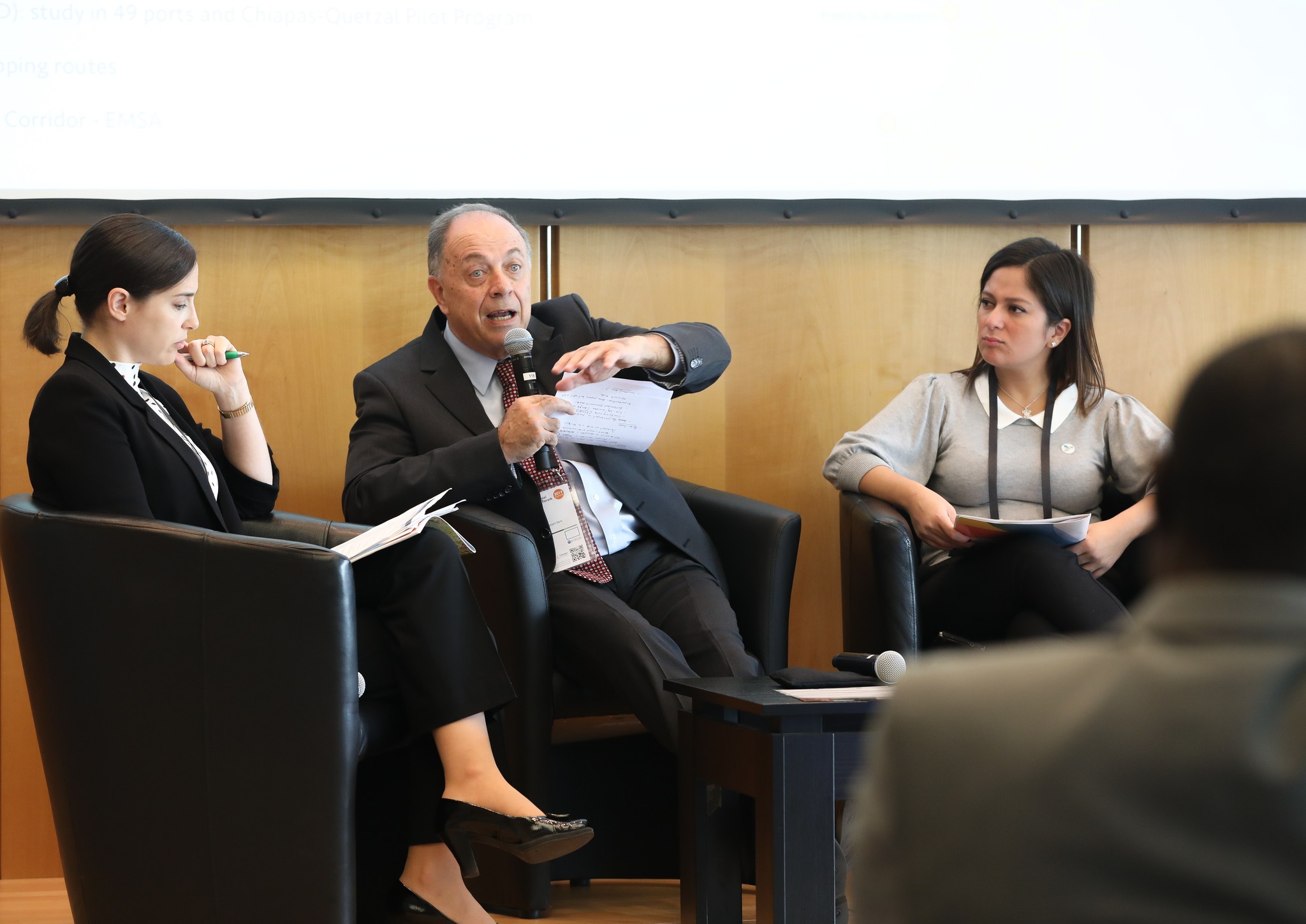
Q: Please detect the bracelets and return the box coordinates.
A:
[217,394,254,419]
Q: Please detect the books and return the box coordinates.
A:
[953,512,1092,548]
[330,488,476,563]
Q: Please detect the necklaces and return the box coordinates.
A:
[994,378,1051,420]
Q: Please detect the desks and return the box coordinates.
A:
[662,671,893,923]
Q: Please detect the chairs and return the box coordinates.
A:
[840,487,1146,661]
[451,476,803,916]
[1,492,382,923]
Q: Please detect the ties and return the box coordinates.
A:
[495,357,613,585]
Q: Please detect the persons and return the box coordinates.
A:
[341,201,845,913]
[824,235,1175,648]
[21,214,598,923]
[838,321,1306,924]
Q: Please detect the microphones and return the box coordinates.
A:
[505,329,558,471]
[829,650,907,684]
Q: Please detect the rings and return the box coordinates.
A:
[201,339,215,348]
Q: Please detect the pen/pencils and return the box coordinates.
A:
[182,351,250,362]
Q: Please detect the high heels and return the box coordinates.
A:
[392,877,460,924]
[438,795,595,879]
